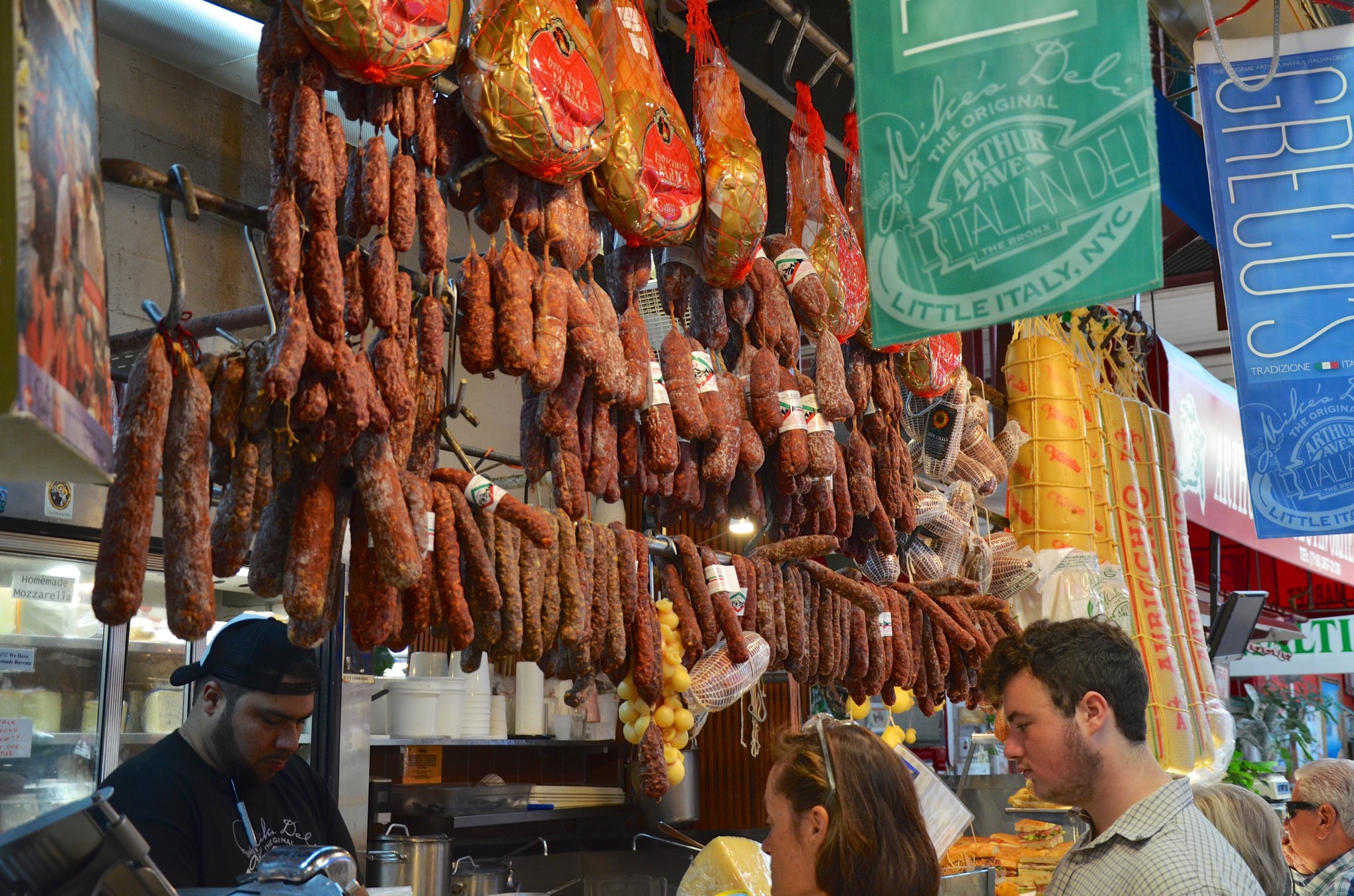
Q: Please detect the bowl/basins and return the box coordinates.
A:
[547,676,574,714]
[407,650,492,738]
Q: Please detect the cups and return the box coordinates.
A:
[568,707,587,740]
[491,695,508,740]
[554,714,572,740]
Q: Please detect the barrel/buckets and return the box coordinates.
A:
[451,855,516,896]
[587,694,619,740]
[365,823,452,896]
[623,731,701,829]
[370,676,467,740]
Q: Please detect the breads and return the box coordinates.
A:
[1007,778,1073,809]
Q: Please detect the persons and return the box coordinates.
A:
[1280,757,1354,896]
[976,618,1269,896]
[761,712,940,896]
[95,614,365,896]
[1190,784,1294,896]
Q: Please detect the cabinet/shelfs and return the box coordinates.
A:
[0,634,186,744]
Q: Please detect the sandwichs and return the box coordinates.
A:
[940,818,1076,896]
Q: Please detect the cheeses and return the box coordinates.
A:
[0,565,98,638]
[129,616,155,641]
[81,699,128,733]
[0,689,61,732]
[676,836,773,896]
[141,689,184,733]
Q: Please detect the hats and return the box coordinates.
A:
[169,617,322,694]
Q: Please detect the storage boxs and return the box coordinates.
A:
[389,783,531,818]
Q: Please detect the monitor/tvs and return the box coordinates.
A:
[1206,590,1269,664]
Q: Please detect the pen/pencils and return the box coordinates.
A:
[231,777,258,846]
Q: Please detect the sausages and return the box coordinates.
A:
[91,1,1038,801]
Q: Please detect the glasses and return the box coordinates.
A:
[1287,801,1341,820]
[801,712,837,790]
